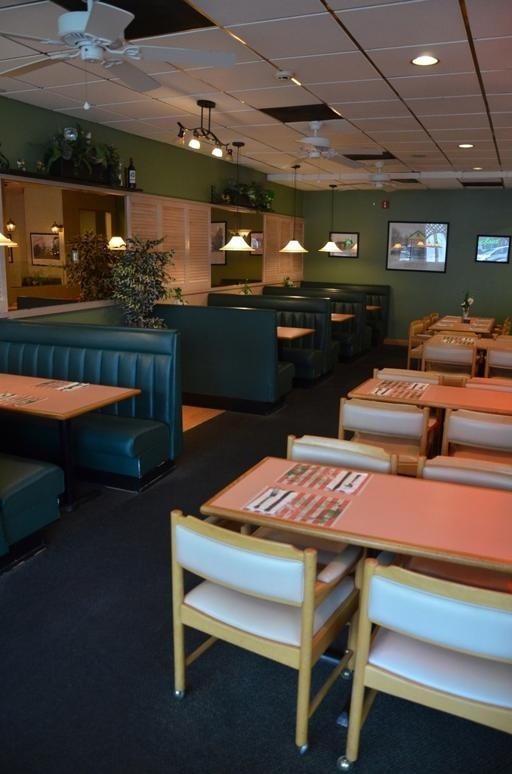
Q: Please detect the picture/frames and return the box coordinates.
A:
[476,233,511,264]
[210,221,226,264]
[4,234,13,264]
[329,231,359,259]
[250,231,263,256]
[386,221,449,274]
[30,232,62,268]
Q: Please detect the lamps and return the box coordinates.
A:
[210,144,224,158]
[0,232,18,246]
[278,167,309,252]
[50,221,62,234]
[6,218,16,235]
[220,148,256,251]
[79,46,104,111]
[317,186,345,253]
[228,229,252,237]
[187,130,201,149]
[104,236,126,251]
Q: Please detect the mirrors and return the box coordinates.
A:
[212,207,263,288]
[0,173,126,320]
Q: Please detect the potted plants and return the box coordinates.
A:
[460,290,473,323]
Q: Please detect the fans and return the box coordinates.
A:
[1,0,232,93]
[246,122,382,170]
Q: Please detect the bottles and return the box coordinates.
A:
[127,158,136,192]
[35,159,45,177]
[17,159,28,173]
